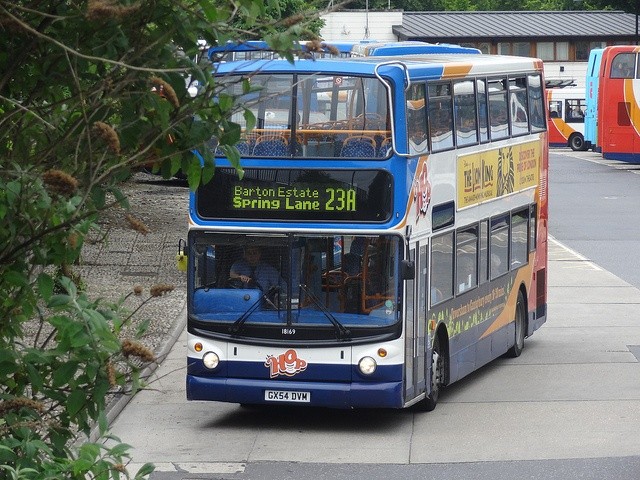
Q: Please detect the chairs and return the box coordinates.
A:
[342,232,534,304]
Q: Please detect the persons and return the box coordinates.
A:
[572,106,583,118]
[613,62,631,78]
[230,247,288,295]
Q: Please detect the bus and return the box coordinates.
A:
[204,39,483,57]
[539,80,586,151]
[596,45,639,164]
[174,40,550,412]
[584,47,604,154]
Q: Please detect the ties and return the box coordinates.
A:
[249,266,257,287]
